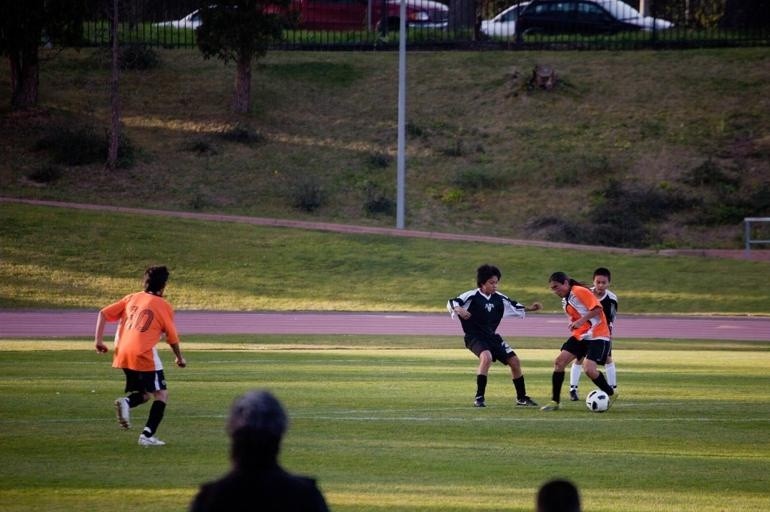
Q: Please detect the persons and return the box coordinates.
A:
[569,266,620,404]
[188,387,331,512]
[92,264,188,450]
[446,264,543,408]
[541,271,621,412]
[537,478,582,511]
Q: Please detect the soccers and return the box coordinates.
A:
[587,390,611,413]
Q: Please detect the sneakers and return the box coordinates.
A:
[138,434,164,446]
[115,397,130,429]
[474,400,486,408]
[571,390,578,401]
[541,402,558,410]
[515,397,536,406]
[608,392,619,411]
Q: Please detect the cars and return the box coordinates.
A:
[255,0,451,34]
[479,0,677,41]
[150,3,239,35]
